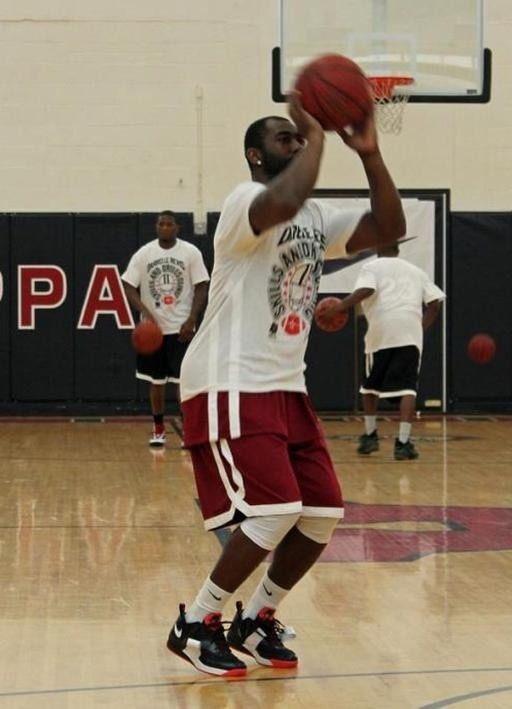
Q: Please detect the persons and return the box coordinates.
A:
[317,238,446,461]
[120,211,211,447]
[165,76,405,678]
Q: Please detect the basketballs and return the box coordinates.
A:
[133,322,163,354]
[293,54,372,131]
[315,298,348,330]
[468,334,496,363]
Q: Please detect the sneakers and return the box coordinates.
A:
[227,601,298,668]
[148,423,167,444]
[394,438,418,460]
[167,604,246,677]
[358,429,379,454]
